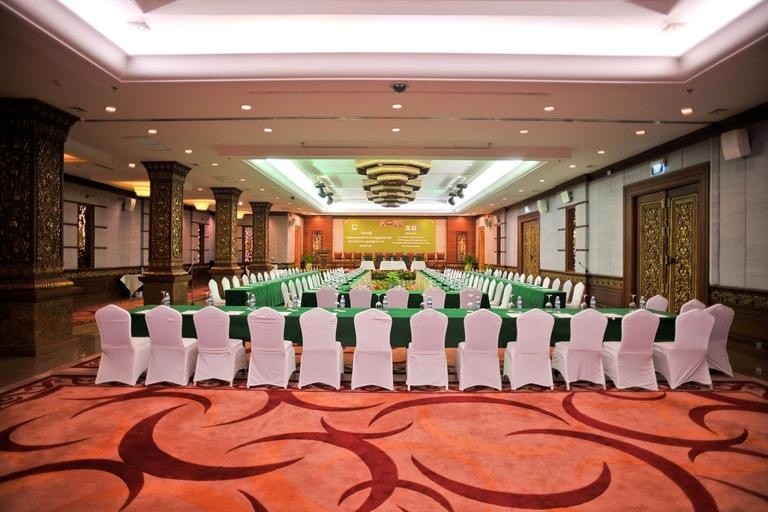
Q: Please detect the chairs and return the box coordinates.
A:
[406,309,449,391]
[443,268,490,294]
[460,289,483,309]
[281,283,301,307]
[568,282,585,309]
[552,278,561,292]
[316,286,339,308]
[704,303,736,378]
[246,307,296,389]
[193,306,248,387]
[486,267,541,287]
[232,275,240,288]
[503,308,555,391]
[498,283,513,308]
[487,279,497,302]
[603,308,659,392]
[553,308,607,391]
[349,287,372,307]
[222,277,231,296]
[308,283,314,290]
[242,274,249,286]
[299,308,345,391]
[492,281,504,308]
[423,286,447,308]
[350,308,393,391]
[386,286,409,308]
[454,309,502,391]
[296,283,303,300]
[654,310,716,390]
[646,295,668,312]
[679,298,705,314]
[313,267,346,286]
[95,304,151,387]
[145,305,198,386]
[563,279,573,303]
[209,279,226,304]
[542,277,550,288]
[250,267,313,284]
[288,283,302,304]
[303,283,308,292]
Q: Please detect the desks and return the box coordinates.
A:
[339,287,350,308]
[372,289,492,309]
[302,292,317,308]
[380,261,406,271]
[121,274,144,295]
[127,304,677,342]
[347,270,372,284]
[411,261,426,271]
[513,288,567,308]
[361,261,375,272]
[225,284,284,307]
[418,269,443,288]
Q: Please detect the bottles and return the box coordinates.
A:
[639,297,645,310]
[423,266,546,292]
[235,265,364,289]
[554,296,560,313]
[589,296,595,309]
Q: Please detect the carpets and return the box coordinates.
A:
[1,347,768,510]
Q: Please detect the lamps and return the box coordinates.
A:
[447,182,468,206]
[361,179,421,199]
[352,157,430,187]
[366,191,417,208]
[133,185,151,198]
[191,201,210,213]
[313,180,335,206]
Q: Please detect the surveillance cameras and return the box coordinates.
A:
[392,82,408,93]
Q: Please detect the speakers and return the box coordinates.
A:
[537,200,548,212]
[560,191,571,203]
[123,198,136,212]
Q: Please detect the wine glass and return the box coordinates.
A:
[545,294,552,313]
[580,294,587,310]
[160,293,522,312]
[628,295,636,312]
